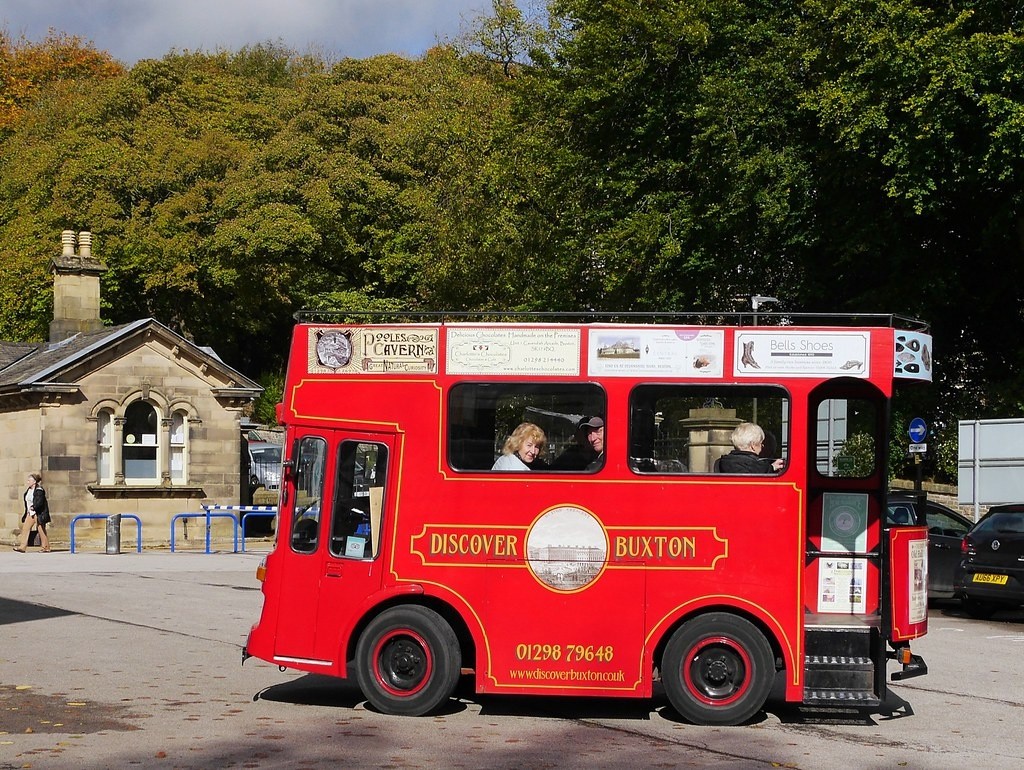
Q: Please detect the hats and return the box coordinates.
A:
[579,415,604,429]
[30,474,41,483]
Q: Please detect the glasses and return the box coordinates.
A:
[757,443,764,448]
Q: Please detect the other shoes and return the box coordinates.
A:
[13,548,25,553]
[39,549,51,553]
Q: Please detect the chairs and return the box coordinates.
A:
[894,508,908,526]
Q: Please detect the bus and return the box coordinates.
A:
[236,294,937,727]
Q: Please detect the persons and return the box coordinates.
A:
[13,473,52,553]
[759,429,778,458]
[712,423,786,473]
[491,422,548,472]
[579,414,640,472]
[552,416,598,470]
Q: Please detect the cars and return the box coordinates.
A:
[952,503,1024,621]
[246,439,283,492]
[885,493,976,608]
